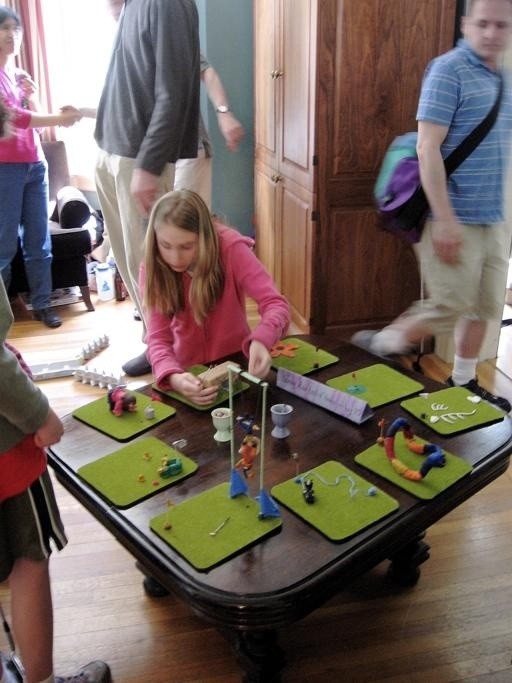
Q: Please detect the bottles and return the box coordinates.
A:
[95,262,115,302]
[114,265,126,301]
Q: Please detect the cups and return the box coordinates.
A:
[270,404,294,438]
[211,408,233,442]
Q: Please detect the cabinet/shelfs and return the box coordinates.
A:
[256,1,457,354]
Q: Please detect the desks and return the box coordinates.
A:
[44,333,512,683]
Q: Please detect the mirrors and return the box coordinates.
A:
[231,365,265,507]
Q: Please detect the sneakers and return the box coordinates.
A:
[122,347,152,376]
[445,376,511,413]
[31,307,62,327]
[54,659,109,683]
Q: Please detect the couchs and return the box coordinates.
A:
[0,141,98,313]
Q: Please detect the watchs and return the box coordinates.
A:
[215,104,231,113]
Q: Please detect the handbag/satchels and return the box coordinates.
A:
[369,64,510,232]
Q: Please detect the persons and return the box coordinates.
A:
[351,1,511,413]
[139,189,292,406]
[0,274,111,682]
[109,0,246,321]
[1,5,81,328]
[60,0,202,378]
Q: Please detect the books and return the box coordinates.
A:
[18,286,85,311]
